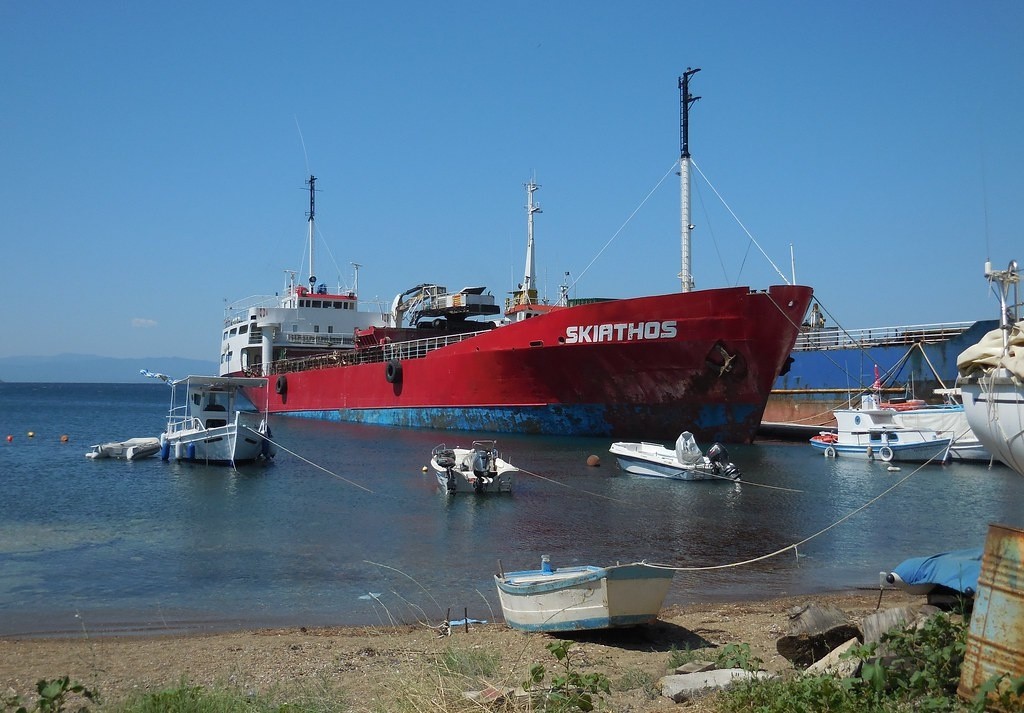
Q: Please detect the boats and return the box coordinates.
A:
[86,437,161,461]
[140,343,269,467]
[758,177,1024,475]
[609,431,743,481]
[886,548,985,611]
[493,555,676,632]
[430,440,520,493]
[219,68,814,445]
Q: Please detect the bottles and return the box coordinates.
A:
[540,555,553,576]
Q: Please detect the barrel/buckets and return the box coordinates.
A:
[956,522,1024,713]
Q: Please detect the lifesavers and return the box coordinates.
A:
[879,447,894,461]
[386,359,401,383]
[825,446,836,458]
[275,376,286,394]
